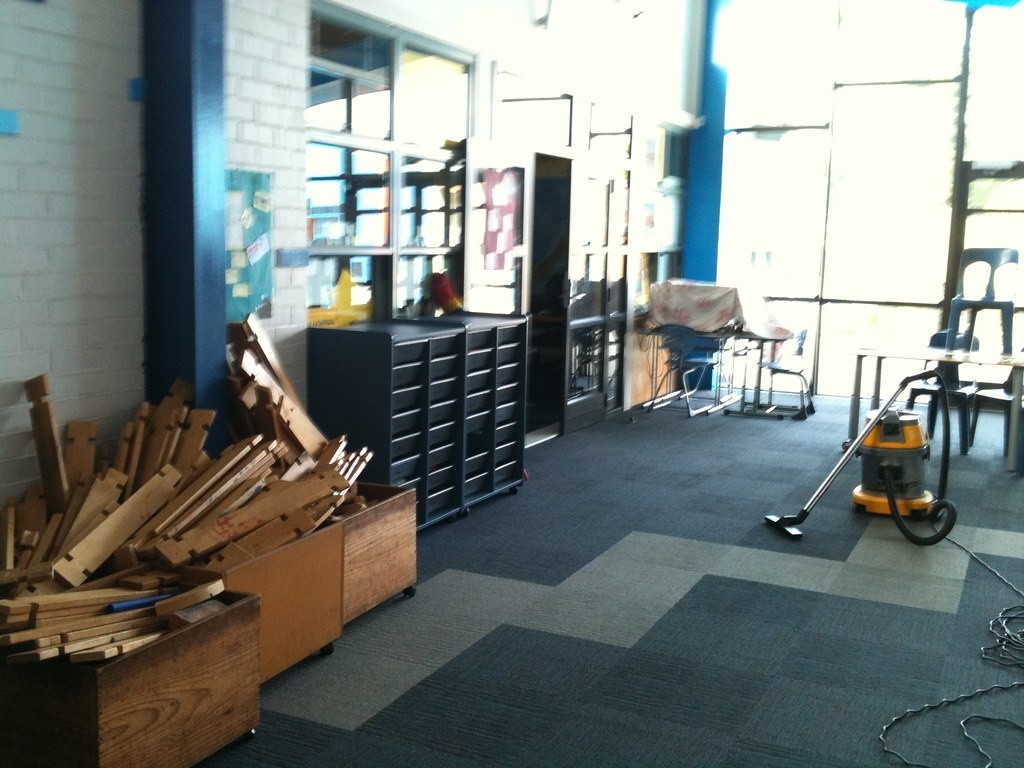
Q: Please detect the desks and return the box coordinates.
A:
[842,343,1024,479]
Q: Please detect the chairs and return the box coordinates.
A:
[759,327,817,424]
[648,330,736,416]
[945,248,1020,355]
[906,331,979,456]
[967,348,1024,458]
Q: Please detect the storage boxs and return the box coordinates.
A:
[331,486,418,625]
[0,587,263,768]
[188,520,344,686]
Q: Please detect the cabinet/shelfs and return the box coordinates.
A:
[398,309,528,513]
[309,320,465,529]
